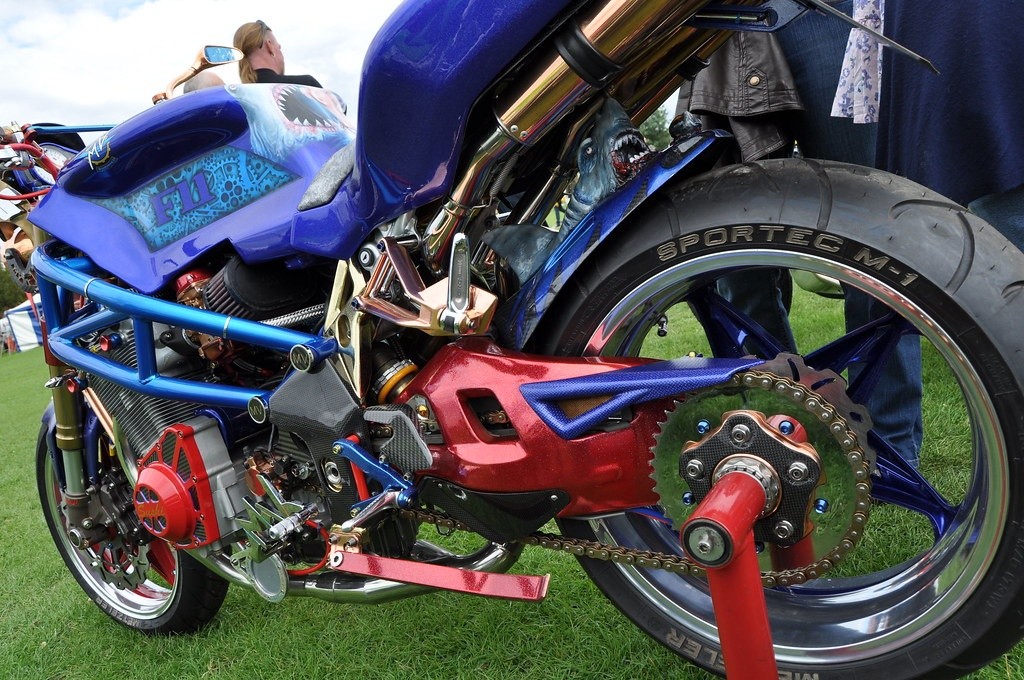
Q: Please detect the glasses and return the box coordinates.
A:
[256,20,271,50]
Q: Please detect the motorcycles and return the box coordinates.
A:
[0,1,1023,679]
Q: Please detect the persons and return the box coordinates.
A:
[232,19,324,89]
[677,0,923,472]
[1,331,9,352]
